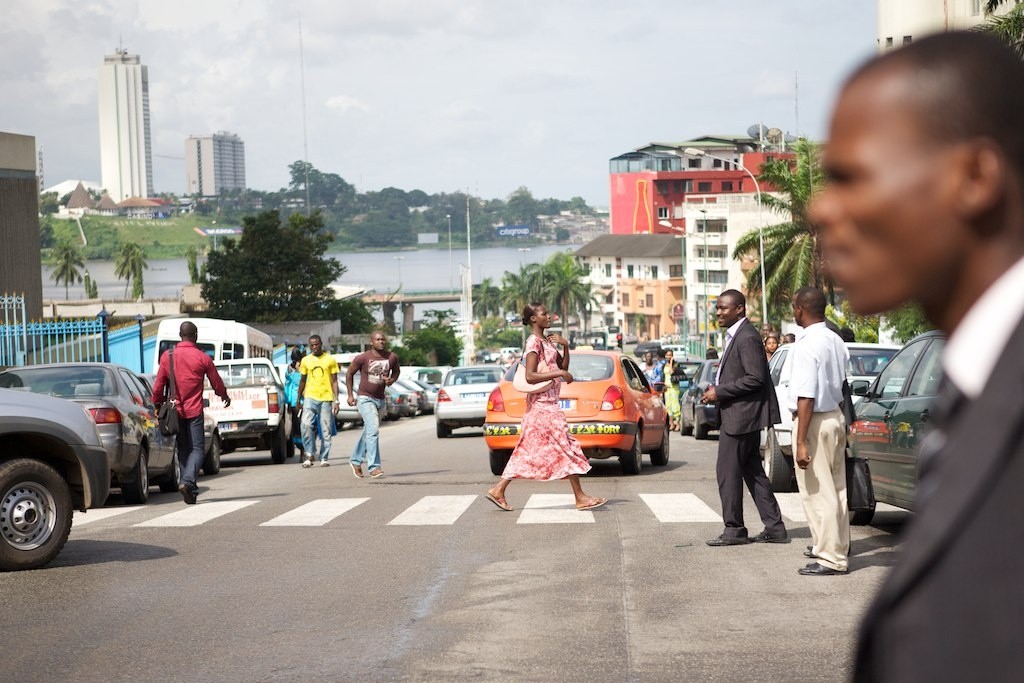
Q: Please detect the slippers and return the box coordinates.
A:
[485,492,514,511]
[576,495,608,511]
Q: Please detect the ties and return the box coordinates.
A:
[913,370,969,479]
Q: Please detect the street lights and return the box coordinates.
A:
[684,148,768,341]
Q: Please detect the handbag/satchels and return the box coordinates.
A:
[671,370,688,383]
[157,399,180,436]
[512,360,554,393]
[846,456,876,511]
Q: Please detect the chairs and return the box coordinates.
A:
[454,373,498,384]
[50,381,104,396]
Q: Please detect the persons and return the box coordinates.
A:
[894,420,912,449]
[640,348,682,433]
[808,28,1024,683]
[700,289,787,547]
[486,302,609,512]
[346,331,401,479]
[700,286,867,577]
[152,323,231,505]
[282,335,341,467]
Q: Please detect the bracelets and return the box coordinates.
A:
[334,400,340,404]
[564,354,569,357]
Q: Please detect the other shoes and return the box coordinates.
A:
[299,455,305,462]
[705,534,748,546]
[179,482,200,504]
[752,530,786,541]
[804,545,851,558]
[797,563,849,575]
[349,460,364,478]
[303,459,312,468]
[670,425,680,431]
[370,468,384,478]
[320,461,330,467]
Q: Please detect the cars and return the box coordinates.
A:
[434,365,506,438]
[483,350,670,475]
[0,362,220,571]
[679,330,948,526]
[336,374,436,429]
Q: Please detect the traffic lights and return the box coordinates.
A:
[617,332,623,348]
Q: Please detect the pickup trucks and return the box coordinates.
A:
[203,357,295,463]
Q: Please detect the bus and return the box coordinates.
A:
[153,318,273,382]
